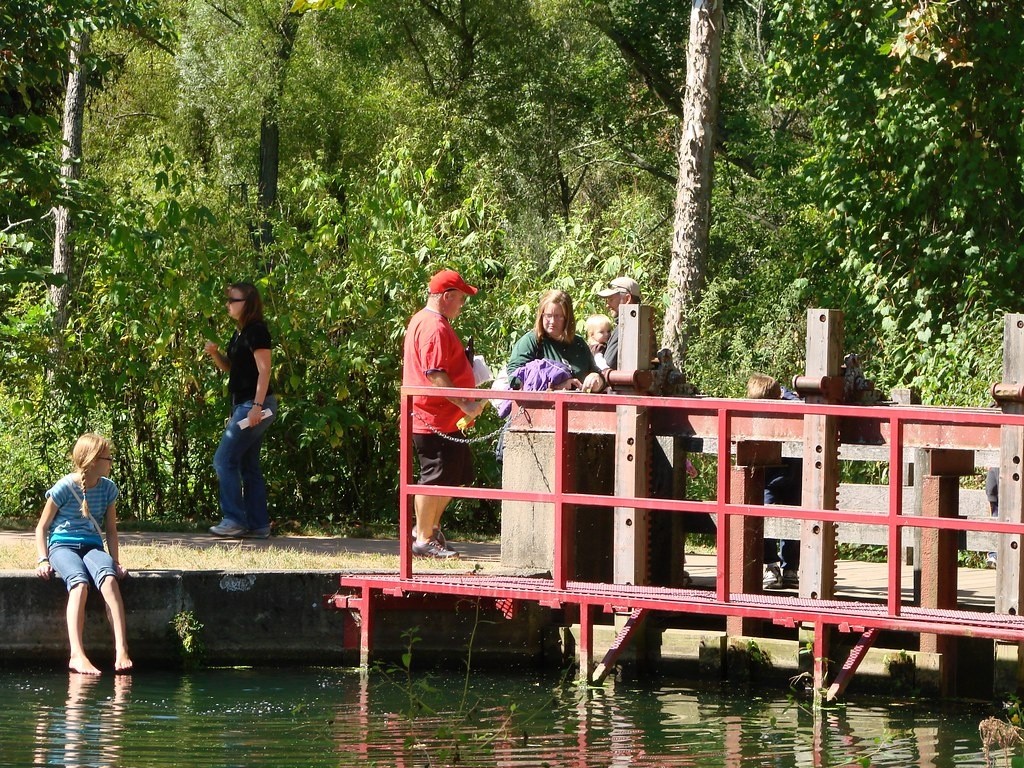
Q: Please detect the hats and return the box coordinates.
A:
[597,277,641,297]
[429,270,478,296]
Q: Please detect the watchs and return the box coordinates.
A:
[37,555,47,564]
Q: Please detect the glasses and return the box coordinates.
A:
[227,298,245,303]
[608,283,632,296]
[541,313,566,322]
[98,457,113,465]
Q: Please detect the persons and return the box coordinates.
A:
[402,269,484,561]
[36,432,132,675]
[204,282,278,539]
[508,276,803,587]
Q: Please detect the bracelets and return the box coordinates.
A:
[252,402,262,407]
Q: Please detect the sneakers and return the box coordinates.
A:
[412,523,446,548]
[209,519,245,537]
[412,535,459,559]
[763,565,783,589]
[239,529,271,537]
[782,570,799,589]
